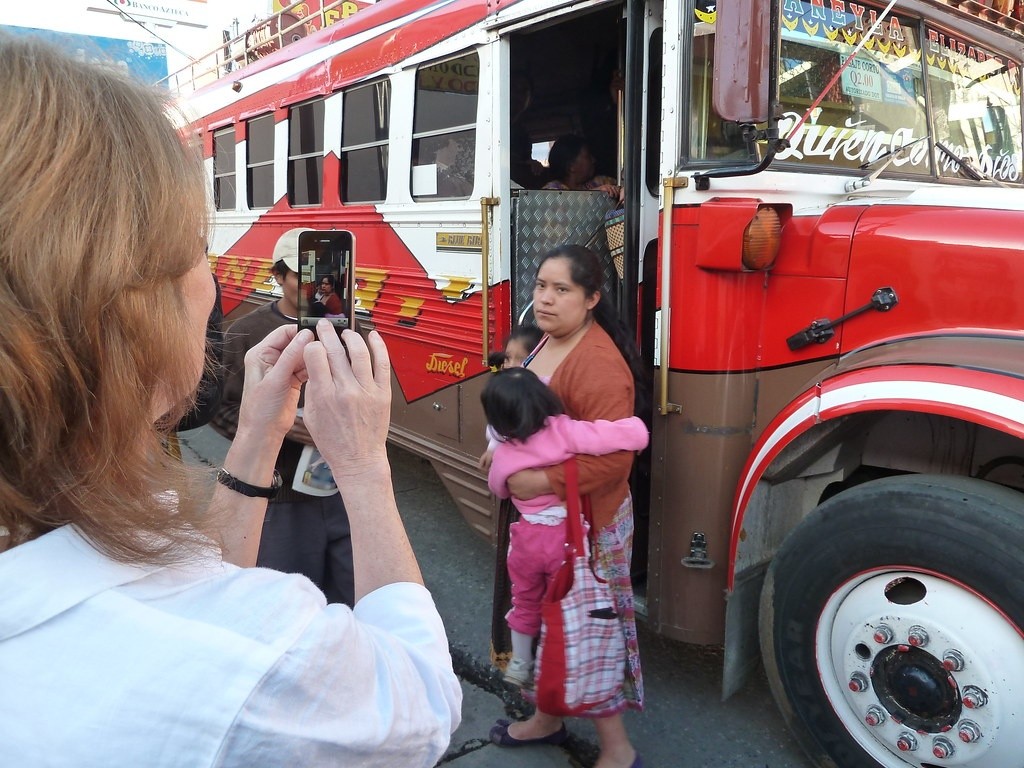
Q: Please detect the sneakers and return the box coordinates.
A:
[503,657,529,687]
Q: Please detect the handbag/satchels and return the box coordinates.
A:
[605,197,625,284]
[531,455,626,715]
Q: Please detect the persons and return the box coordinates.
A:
[489,244,647,768]
[481,367,648,691]
[148,274,226,519]
[511,66,623,199]
[207,224,355,611]
[503,325,545,368]
[0,28,464,768]
[305,273,346,320]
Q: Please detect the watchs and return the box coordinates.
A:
[217,467,283,498]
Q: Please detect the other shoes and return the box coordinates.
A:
[628,746,641,768]
[490,719,567,747]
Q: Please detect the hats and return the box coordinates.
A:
[272,228,317,273]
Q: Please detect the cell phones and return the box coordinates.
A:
[297,229,356,347]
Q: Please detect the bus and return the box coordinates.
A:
[152,0,1024,767]
